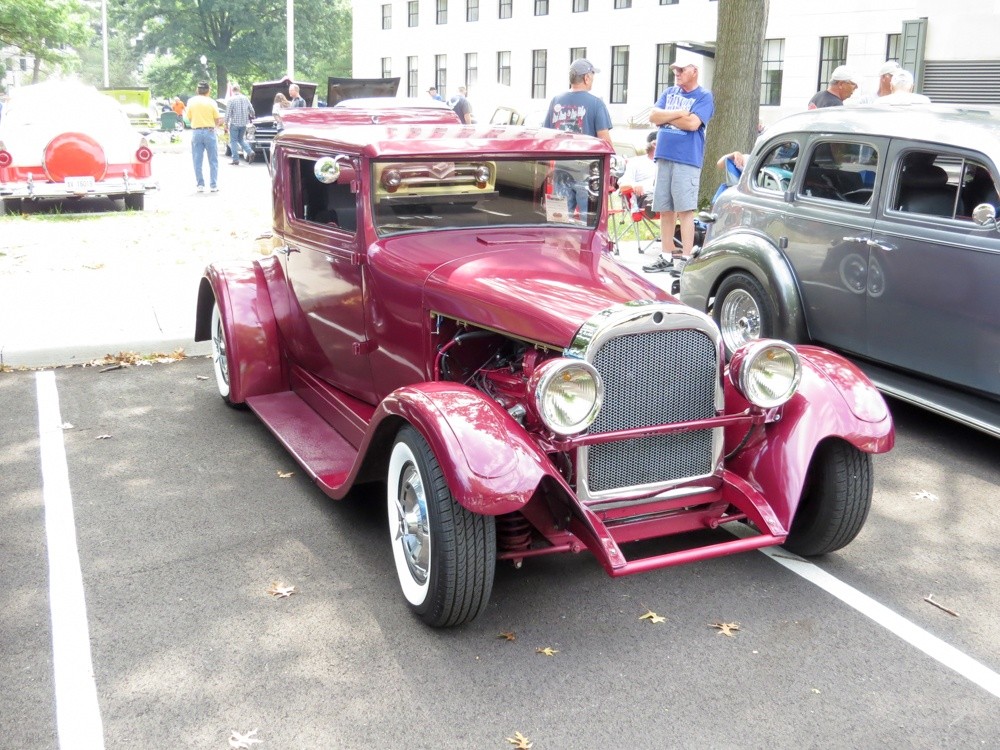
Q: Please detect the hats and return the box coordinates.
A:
[570,58,600,75]
[671,56,701,68]
[832,65,863,88]
[425,87,436,92]
[198,80,213,88]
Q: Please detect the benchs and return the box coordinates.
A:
[800,168,876,205]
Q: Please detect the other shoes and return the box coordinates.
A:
[197,185,205,192]
[211,187,220,191]
[248,151,255,164]
[229,160,240,165]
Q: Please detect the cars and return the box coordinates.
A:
[196,101,896,631]
[667,108,999,451]
[0,76,161,217]
[237,82,319,163]
[310,75,407,113]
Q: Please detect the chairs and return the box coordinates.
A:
[902,166,963,219]
[330,205,398,233]
[968,166,1000,218]
[612,186,683,254]
[609,185,625,255]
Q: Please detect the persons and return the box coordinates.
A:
[617,131,657,219]
[873,60,931,105]
[717,151,750,173]
[224,85,256,165]
[643,60,714,273]
[186,81,220,192]
[426,87,441,100]
[161,96,185,128]
[446,87,471,124]
[544,58,613,224]
[795,65,859,157]
[272,84,306,133]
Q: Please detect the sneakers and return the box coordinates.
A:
[642,254,674,273]
[670,257,688,278]
